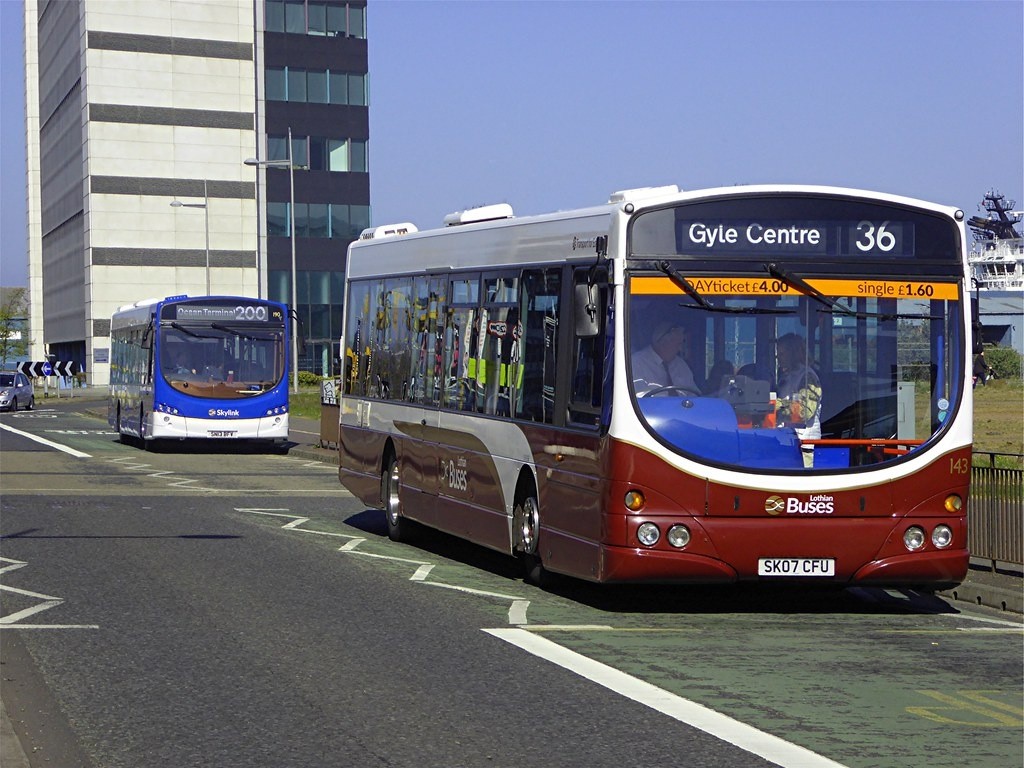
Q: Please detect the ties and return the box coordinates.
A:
[662,361,678,396]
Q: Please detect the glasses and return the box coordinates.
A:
[658,322,683,341]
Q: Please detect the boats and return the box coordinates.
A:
[967,189,1024,291]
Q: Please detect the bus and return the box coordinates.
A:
[338,181,980,595]
[108,295,306,452]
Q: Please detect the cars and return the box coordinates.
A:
[0,371,34,412]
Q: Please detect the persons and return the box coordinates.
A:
[776,333,822,469]
[631,320,703,396]
[171,351,199,374]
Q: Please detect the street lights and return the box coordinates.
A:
[244,156,298,392]
[170,201,209,295]
